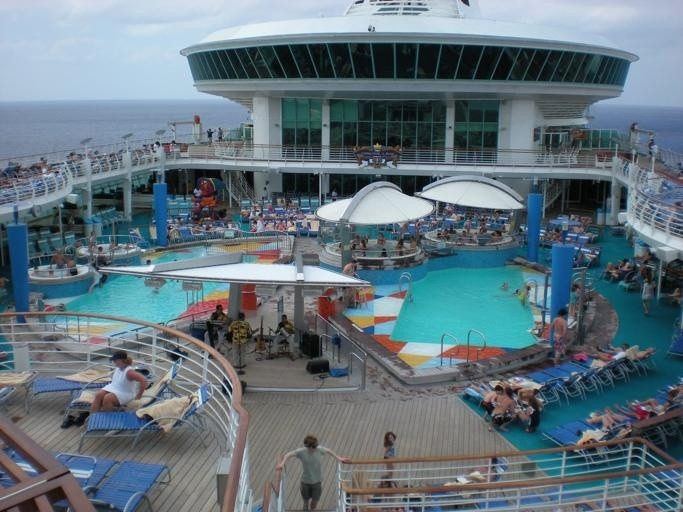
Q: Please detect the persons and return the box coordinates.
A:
[441,456,497,501]
[381,431,396,483]
[598,342,657,360]
[226,312,255,369]
[0,139,181,214]
[215,127,223,142]
[468,375,545,435]
[205,304,230,350]
[585,383,683,433]
[628,121,655,165]
[327,204,602,258]
[673,163,682,179]
[90,349,147,412]
[342,260,361,309]
[49,230,153,288]
[646,138,656,159]
[550,310,568,369]
[274,434,351,511]
[516,285,532,308]
[0,274,10,306]
[206,128,213,147]
[268,313,296,358]
[584,342,628,362]
[165,181,320,244]
[570,126,586,158]
[603,247,683,315]
[369,475,398,504]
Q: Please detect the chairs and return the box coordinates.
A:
[1,386,15,403]
[2,447,72,486]
[463,184,681,470]
[77,381,215,452]
[82,461,171,510]
[25,365,155,415]
[60,357,183,429]
[0,368,36,393]
[2,141,462,303]
[55,452,121,511]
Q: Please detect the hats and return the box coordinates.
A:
[109,350,127,361]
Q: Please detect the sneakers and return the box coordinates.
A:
[61,415,75,428]
[73,411,90,427]
